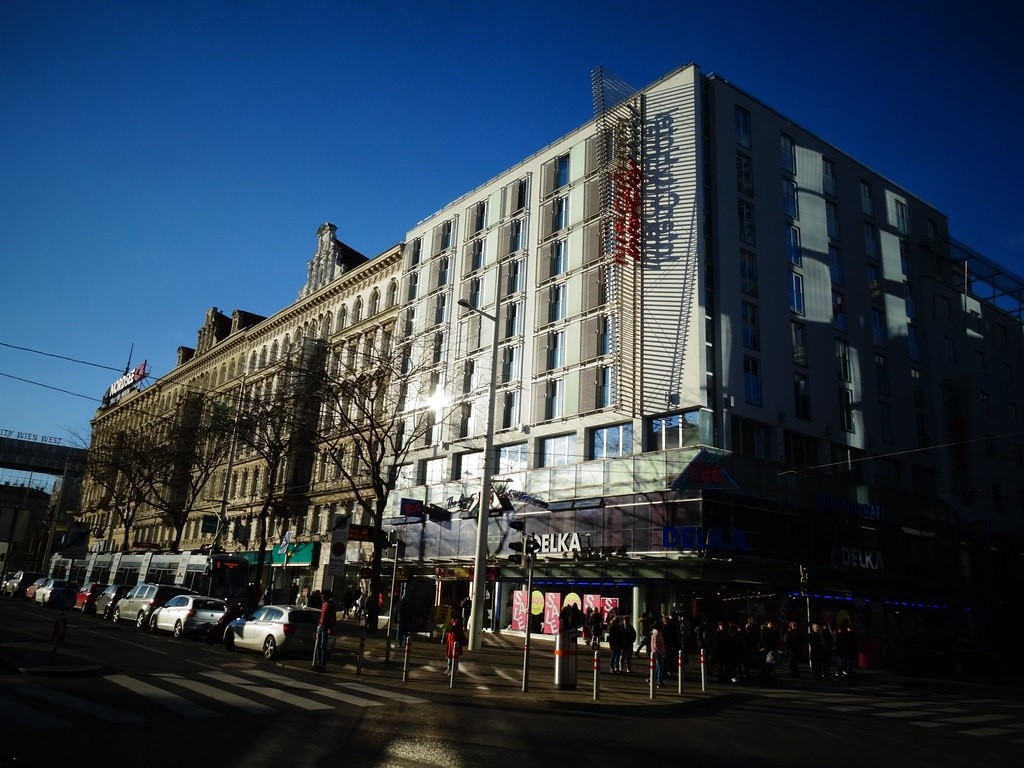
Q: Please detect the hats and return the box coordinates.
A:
[450,618,459,625]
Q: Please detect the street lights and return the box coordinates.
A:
[457,297,500,653]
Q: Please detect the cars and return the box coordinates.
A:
[150,594,230,640]
[25,577,51,599]
[32,579,77,609]
[0,573,15,590]
[222,603,336,662]
[67,584,109,612]
[94,584,135,619]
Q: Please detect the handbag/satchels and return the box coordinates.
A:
[327,633,336,649]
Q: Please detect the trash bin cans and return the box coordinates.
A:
[552,631,579,685]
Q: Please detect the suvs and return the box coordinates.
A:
[3,570,46,599]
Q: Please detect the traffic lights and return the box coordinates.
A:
[802,566,812,595]
[526,540,541,554]
[508,519,525,567]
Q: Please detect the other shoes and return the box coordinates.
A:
[614,670,621,674]
[626,669,633,672]
[657,684,665,688]
[645,678,649,683]
[448,671,458,675]
[619,669,623,672]
[443,669,451,675]
[608,669,615,674]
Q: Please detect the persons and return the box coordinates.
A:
[634,610,857,689]
[308,590,322,609]
[442,605,463,677]
[310,589,336,673]
[339,587,368,621]
[463,596,472,629]
[264,586,298,605]
[394,595,413,648]
[560,603,604,650]
[609,615,636,673]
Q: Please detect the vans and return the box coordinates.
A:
[112,583,201,629]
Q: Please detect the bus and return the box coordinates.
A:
[45,552,251,603]
